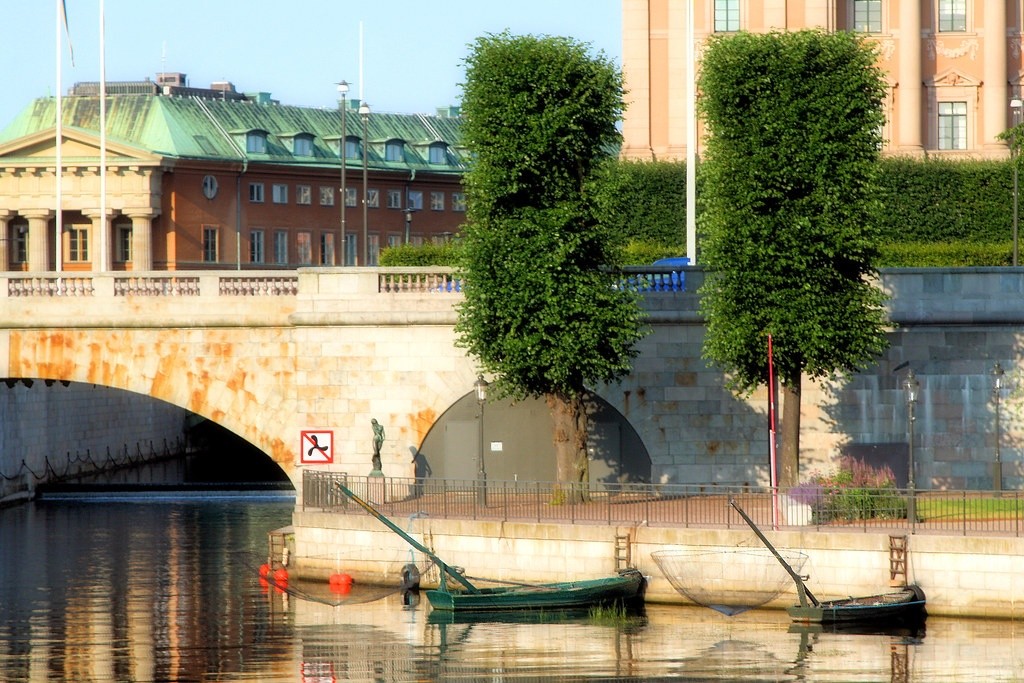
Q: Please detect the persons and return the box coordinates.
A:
[370,418,386,455]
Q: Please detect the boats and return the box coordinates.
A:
[336,480,642,612]
[731,497,928,635]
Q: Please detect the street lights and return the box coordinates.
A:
[474,375,490,504]
[989,362,1005,489]
[357,101,371,268]
[400,208,417,244]
[1009,90,1022,267]
[334,79,352,266]
[903,368,920,533]
